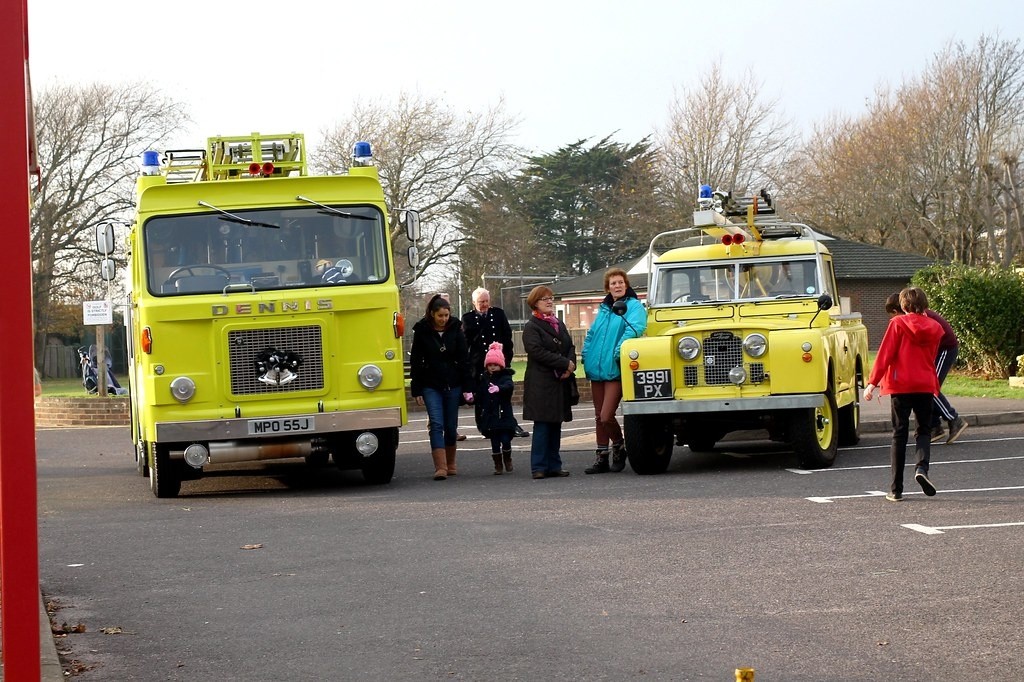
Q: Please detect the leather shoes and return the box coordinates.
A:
[532,469,570,478]
[513,424,530,437]
[456,432,466,441]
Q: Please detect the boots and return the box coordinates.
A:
[584,450,611,474]
[492,449,513,475]
[611,439,626,473]
[431,444,457,479]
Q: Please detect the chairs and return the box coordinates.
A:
[175,274,227,292]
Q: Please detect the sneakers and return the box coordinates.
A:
[930,416,968,446]
[886,492,904,501]
[914,468,937,497]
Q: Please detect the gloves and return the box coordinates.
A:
[488,382,499,393]
[463,392,474,402]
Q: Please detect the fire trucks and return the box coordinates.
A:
[96,131,420,496]
[619,186,868,470]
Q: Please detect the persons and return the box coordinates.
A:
[34,368,41,397]
[461,288,530,439]
[885,292,968,444]
[315,260,347,284]
[863,288,945,501]
[467,341,516,475]
[410,293,472,480]
[581,267,648,474]
[522,285,577,479]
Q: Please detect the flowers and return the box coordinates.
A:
[1015,353,1024,375]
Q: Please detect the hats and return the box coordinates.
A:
[484,341,505,368]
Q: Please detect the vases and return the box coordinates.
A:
[1008,376,1024,387]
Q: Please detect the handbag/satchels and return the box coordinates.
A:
[570,372,580,406]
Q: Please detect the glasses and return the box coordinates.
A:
[537,296,554,301]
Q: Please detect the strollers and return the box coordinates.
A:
[77,343,128,396]
